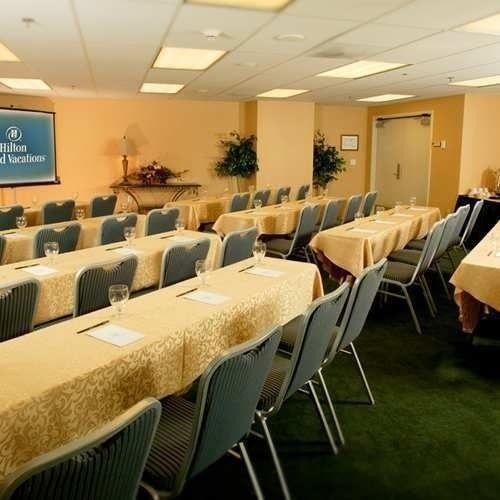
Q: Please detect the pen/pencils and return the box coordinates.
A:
[239,265,254,272]
[488,251,493,256]
[105,246,123,251]
[176,288,197,297]
[2,232,15,235]
[77,321,108,333]
[160,235,174,238]
[15,264,40,269]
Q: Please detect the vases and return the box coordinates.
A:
[143,177,169,185]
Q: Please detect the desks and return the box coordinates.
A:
[1,256,317,486]
[449,219,500,332]
[453,193,500,250]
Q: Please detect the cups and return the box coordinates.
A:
[471,186,496,199]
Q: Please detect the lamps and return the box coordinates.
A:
[105,125,141,182]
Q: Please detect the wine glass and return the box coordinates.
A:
[197,183,272,199]
[42,241,61,267]
[252,241,267,267]
[355,195,418,229]
[174,219,186,239]
[108,284,130,324]
[13,202,128,233]
[251,187,330,213]
[124,227,136,249]
[193,259,213,292]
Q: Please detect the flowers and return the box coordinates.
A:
[134,159,188,177]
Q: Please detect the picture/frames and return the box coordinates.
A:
[340,134,359,151]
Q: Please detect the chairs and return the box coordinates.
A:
[279,256,390,447]
[4,395,158,500]
[224,275,352,500]
[1,186,486,340]
[138,325,285,498]
